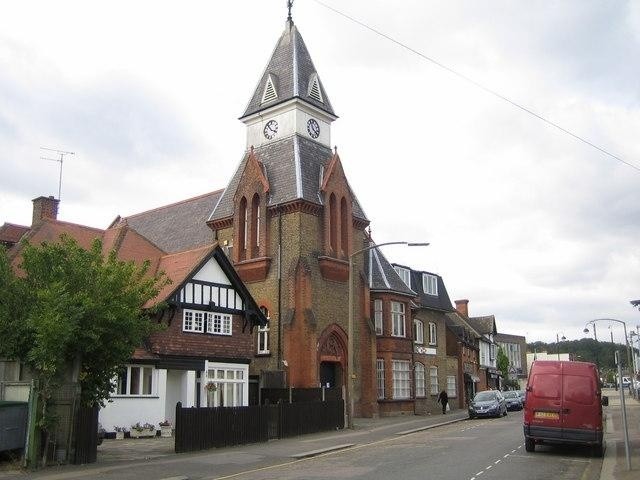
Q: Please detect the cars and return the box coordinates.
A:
[618,376,637,386]
[468,390,525,420]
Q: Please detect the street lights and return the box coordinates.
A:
[583,323,600,373]
[348,241,428,430]
[556,332,566,361]
[584,318,634,398]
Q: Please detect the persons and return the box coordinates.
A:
[438,390,448,414]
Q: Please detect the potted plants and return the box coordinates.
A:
[114,419,173,440]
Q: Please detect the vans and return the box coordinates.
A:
[523,360,608,456]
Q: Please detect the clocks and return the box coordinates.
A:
[306,118,321,139]
[263,119,279,140]
